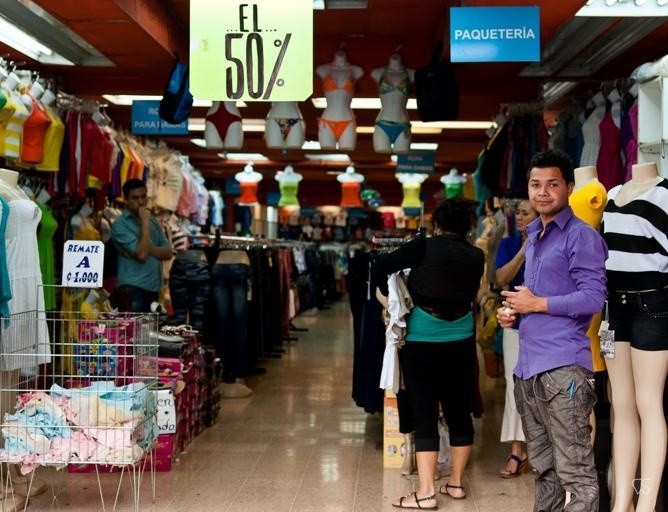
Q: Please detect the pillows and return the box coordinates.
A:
[369,237,417,256]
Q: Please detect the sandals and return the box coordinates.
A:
[438,483,465,500]
[391,492,437,511]
[499,454,527,479]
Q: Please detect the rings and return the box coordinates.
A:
[506,301,514,309]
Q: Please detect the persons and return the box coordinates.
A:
[108,177,173,312]
[395,172,431,209]
[334,166,366,210]
[568,81,640,188]
[210,249,253,386]
[495,146,609,512]
[315,52,366,153]
[600,160,668,512]
[439,168,466,202]
[567,164,612,512]
[493,196,541,480]
[0,57,68,383]
[274,166,303,210]
[370,55,416,155]
[233,165,263,208]
[370,195,486,509]
[167,249,210,342]
[265,102,307,149]
[204,102,245,151]
[69,203,122,240]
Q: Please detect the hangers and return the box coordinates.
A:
[217,237,277,253]
